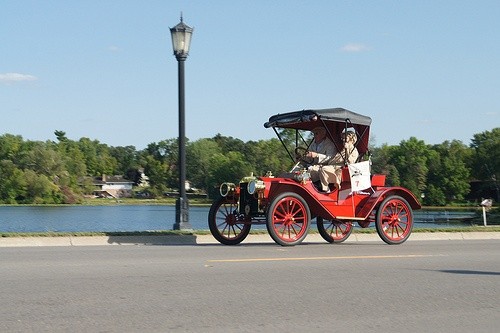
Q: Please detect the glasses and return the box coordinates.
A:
[313,132,319,135]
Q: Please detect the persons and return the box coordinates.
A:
[296,125,337,182]
[308,127,359,194]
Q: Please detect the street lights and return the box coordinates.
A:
[168,9,195,230]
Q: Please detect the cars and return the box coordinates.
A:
[207,107,422,247]
